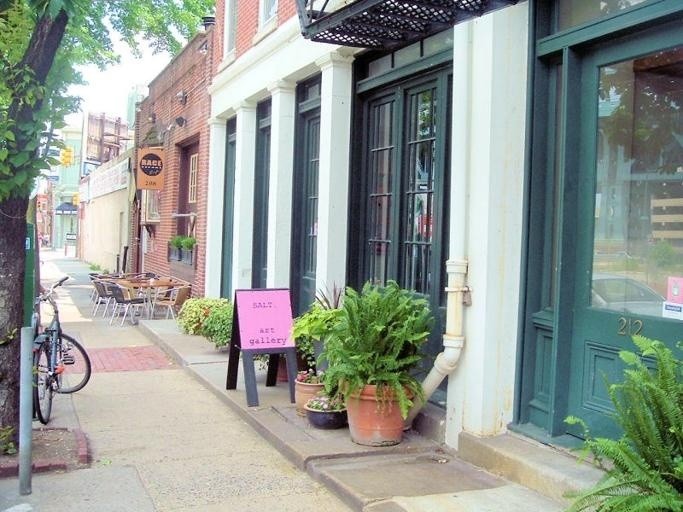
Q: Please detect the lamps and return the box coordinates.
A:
[176,117,187,128]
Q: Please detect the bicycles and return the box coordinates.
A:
[32,276,91,424]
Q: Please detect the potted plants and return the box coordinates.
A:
[253,276,434,448]
[177,297,234,352]
[169,235,196,266]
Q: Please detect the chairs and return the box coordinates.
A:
[89,270,192,326]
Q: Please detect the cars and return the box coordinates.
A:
[590,272,665,317]
